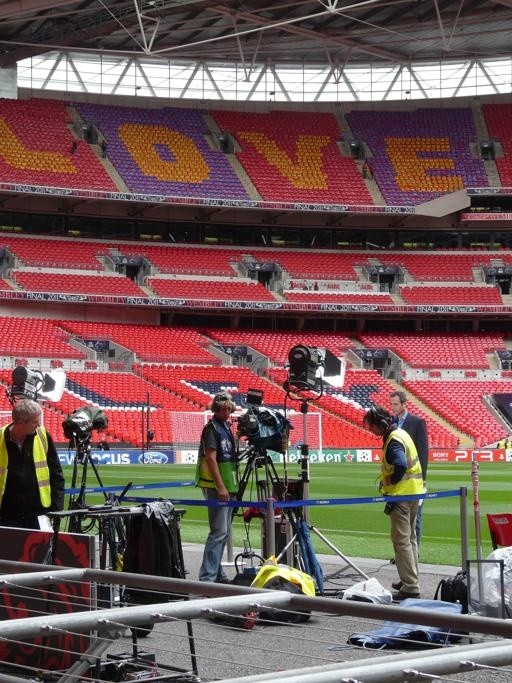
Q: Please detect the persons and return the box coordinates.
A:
[388,392,428,565]
[497,436,511,449]
[364,407,425,599]
[0,399,64,530]
[196,390,239,585]
[98,436,109,449]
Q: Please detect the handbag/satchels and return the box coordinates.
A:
[434,571,468,614]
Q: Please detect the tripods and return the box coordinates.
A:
[269,402,371,589]
[65,446,140,608]
[217,448,326,596]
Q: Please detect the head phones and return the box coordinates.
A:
[370,404,389,431]
[211,396,232,412]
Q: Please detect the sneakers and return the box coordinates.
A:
[393,581,403,589]
[392,590,420,600]
